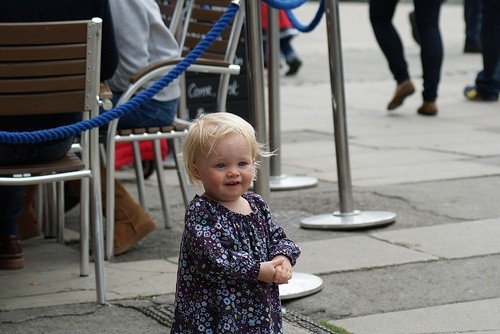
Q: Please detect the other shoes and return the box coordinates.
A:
[286,60,301,75]
[418,101,437,115]
[464,87,497,101]
[0,235,24,268]
[387,80,414,110]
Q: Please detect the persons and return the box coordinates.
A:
[368,0,444,116]
[463,0,500,101]
[260,0,304,76]
[172,112,301,334]
[0,1,120,270]
[89,0,181,259]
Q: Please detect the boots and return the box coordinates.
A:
[17,186,44,243]
[72,164,154,255]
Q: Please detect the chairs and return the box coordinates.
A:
[0,18,105,305]
[71,0,246,261]
